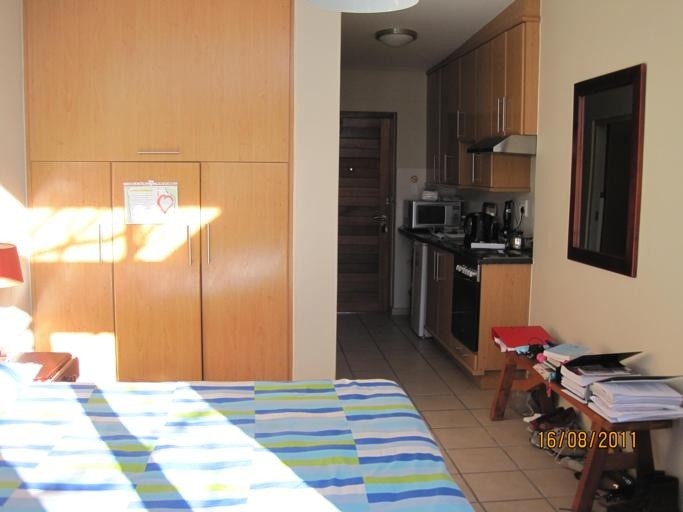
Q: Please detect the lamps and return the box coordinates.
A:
[375,28,418,49]
[0,243,23,288]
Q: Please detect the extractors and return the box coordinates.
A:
[466,135,536,157]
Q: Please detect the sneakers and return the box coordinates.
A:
[522,407,678,512]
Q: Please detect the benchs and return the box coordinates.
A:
[490,346,673,510]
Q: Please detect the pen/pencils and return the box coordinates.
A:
[0,363,21,381]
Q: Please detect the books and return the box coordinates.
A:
[487,321,683,424]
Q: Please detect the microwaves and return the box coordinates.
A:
[406,199,464,231]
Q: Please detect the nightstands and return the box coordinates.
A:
[19,350,78,383]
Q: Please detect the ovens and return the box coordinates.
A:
[452,273,480,353]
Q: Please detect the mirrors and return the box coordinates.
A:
[567,63,646,278]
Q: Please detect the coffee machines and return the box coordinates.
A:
[463,211,496,249]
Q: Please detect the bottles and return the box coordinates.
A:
[508,233,523,250]
[495,201,515,245]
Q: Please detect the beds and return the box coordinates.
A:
[0,357,476,512]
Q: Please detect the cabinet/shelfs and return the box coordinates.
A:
[424,247,454,348]
[423,18,538,194]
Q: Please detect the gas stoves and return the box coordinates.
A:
[452,248,532,281]
